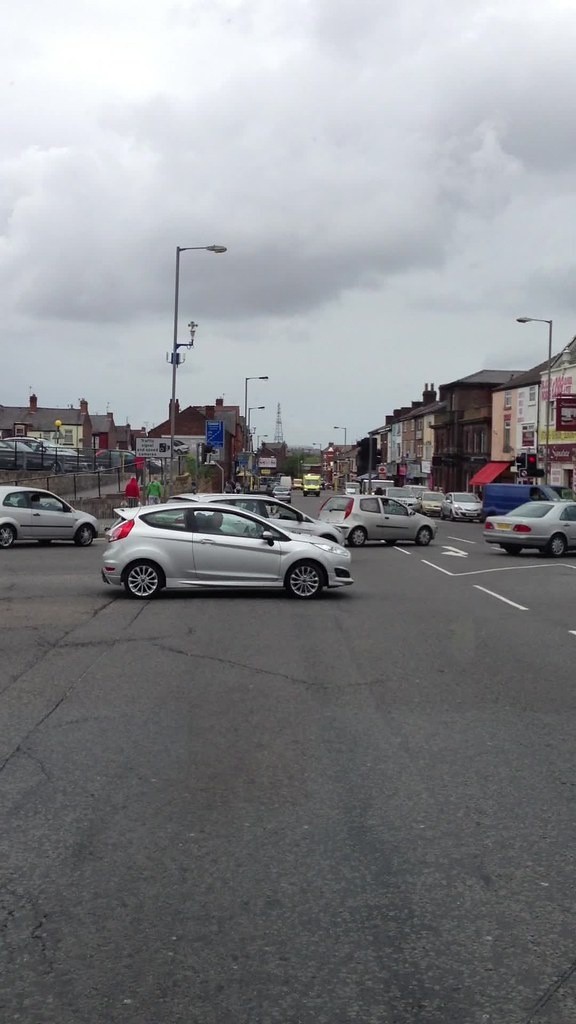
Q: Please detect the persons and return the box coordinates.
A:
[124,476,141,507]
[321,482,326,490]
[147,477,163,505]
[31,495,41,509]
[477,490,483,501]
[133,457,144,485]
[211,512,223,534]
[225,480,241,494]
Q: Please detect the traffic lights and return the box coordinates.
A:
[516,452,526,471]
[526,453,537,477]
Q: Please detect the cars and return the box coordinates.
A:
[382,487,418,511]
[317,494,437,548]
[0,438,86,473]
[0,485,100,547]
[320,481,331,490]
[150,491,346,549]
[270,486,292,503]
[93,449,163,475]
[173,440,190,455]
[101,501,355,600]
[440,492,486,523]
[548,485,576,502]
[482,500,576,558]
[416,492,446,517]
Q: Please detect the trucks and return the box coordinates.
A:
[481,483,561,517]
[301,473,323,497]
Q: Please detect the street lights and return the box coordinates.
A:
[244,376,269,418]
[166,245,228,439]
[333,426,347,461]
[248,406,265,428]
[516,317,553,485]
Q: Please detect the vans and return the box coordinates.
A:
[342,482,360,495]
[403,485,432,501]
[292,478,303,490]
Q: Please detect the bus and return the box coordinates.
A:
[259,472,292,497]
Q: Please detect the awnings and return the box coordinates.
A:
[468,462,511,486]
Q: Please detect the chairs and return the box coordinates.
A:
[196,513,209,532]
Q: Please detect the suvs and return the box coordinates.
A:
[361,480,394,495]
